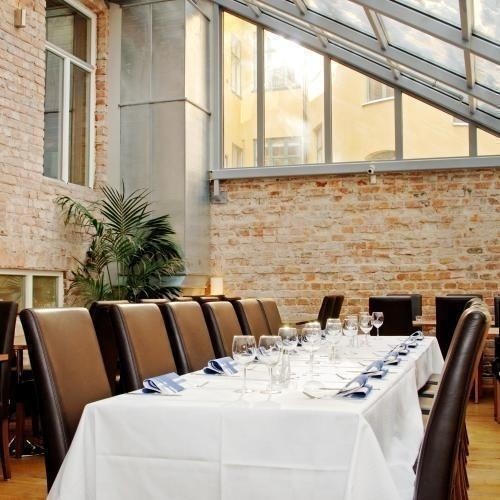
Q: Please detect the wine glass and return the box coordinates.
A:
[232,312,384,396]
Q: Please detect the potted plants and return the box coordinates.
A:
[159,259,187,286]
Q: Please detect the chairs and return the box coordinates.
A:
[0,295,283,500]
[319,293,499,402]
[413,307,491,500]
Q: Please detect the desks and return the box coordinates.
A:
[82,334,435,498]
